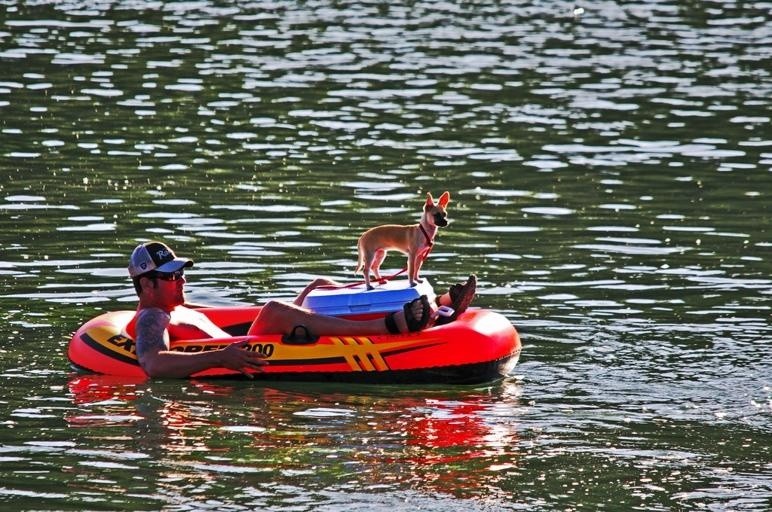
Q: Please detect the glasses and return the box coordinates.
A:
[147,268,183,281]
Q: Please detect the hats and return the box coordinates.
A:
[128,242,193,279]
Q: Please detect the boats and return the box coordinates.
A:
[66,308,521,383]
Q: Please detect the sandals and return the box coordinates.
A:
[385,295,430,334]
[434,274,476,324]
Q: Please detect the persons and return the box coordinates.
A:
[126,242,476,379]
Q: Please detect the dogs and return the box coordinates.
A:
[354,190,450,291]
